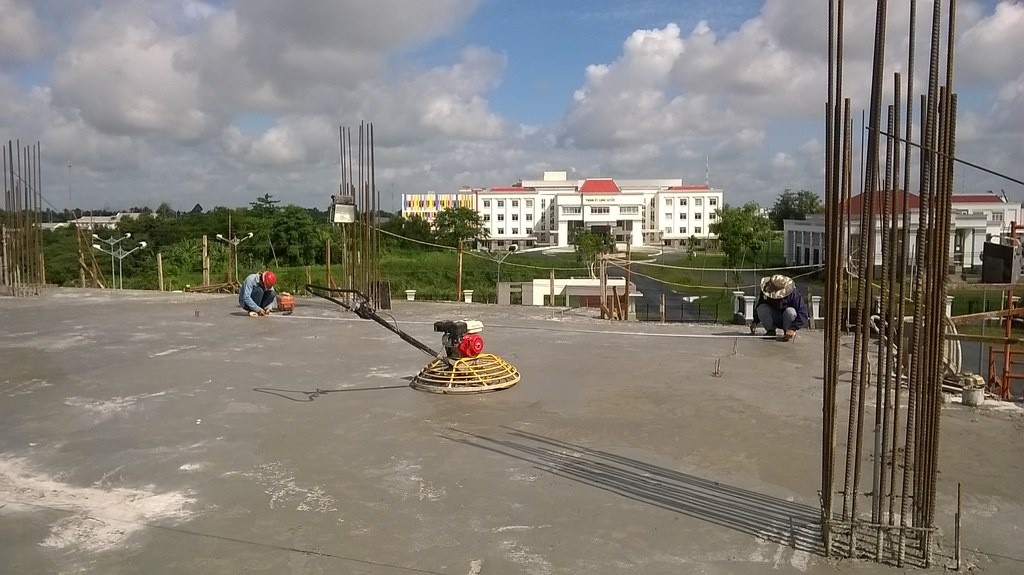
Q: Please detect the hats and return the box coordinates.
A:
[761,274,796,299]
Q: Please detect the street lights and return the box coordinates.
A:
[215,231,254,282]
[91,232,131,290]
[899,239,916,303]
[92,241,148,290]
[480,244,519,282]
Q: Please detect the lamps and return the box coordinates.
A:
[329,195,357,226]
[981,234,1023,284]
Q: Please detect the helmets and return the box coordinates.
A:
[263,271,276,290]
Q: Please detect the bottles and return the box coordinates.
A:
[195,308,199,317]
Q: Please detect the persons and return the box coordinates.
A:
[750,273,809,341]
[238,270,278,317]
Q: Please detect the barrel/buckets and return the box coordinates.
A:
[962,386,985,407]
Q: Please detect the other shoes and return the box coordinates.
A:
[763,329,776,339]
[783,332,796,340]
[249,311,258,317]
